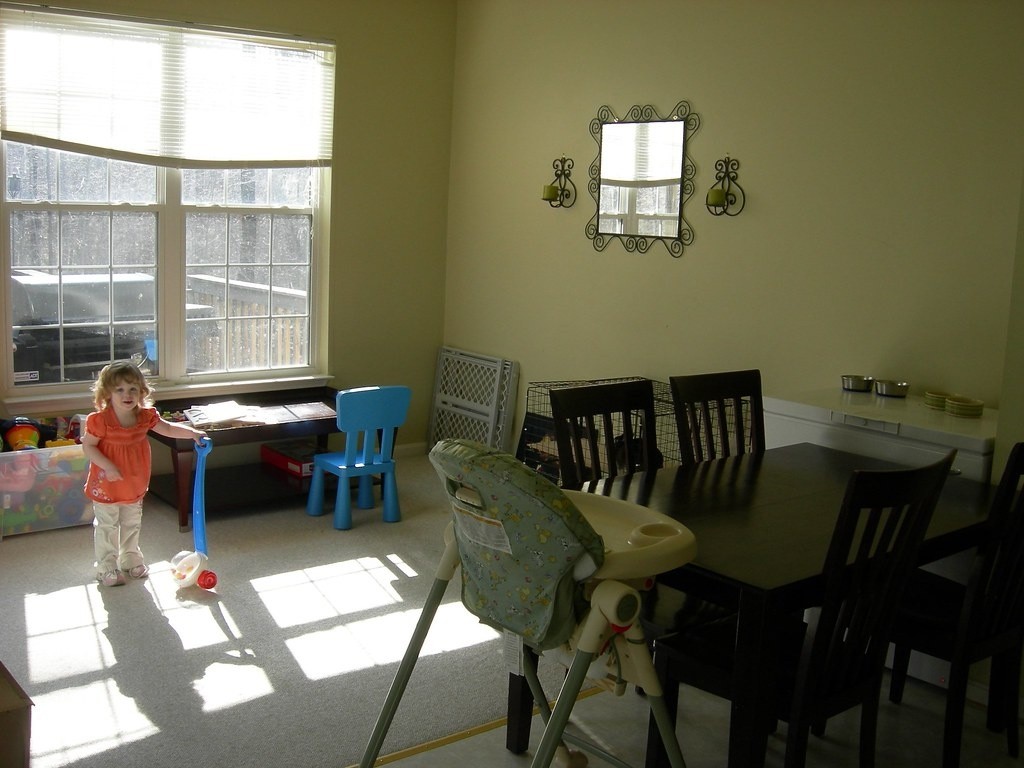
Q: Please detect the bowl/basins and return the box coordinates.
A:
[944,398,985,417]
[841,374,875,392]
[923,390,948,410]
[875,379,911,397]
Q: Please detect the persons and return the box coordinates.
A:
[83,361,208,586]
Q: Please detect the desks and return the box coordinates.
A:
[150,386,398,533]
[507,442,1024,768]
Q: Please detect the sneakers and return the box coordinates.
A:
[96,569,126,587]
[125,563,150,579]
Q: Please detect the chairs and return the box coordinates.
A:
[306,386,413,530]
[357,436,700,768]
[548,367,1024,768]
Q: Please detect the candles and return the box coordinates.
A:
[543,185,558,199]
[707,187,726,205]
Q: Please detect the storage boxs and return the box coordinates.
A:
[0,409,95,540]
[260,439,332,478]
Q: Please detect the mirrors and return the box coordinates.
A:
[597,120,684,240]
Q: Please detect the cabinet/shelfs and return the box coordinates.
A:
[763,388,999,702]
